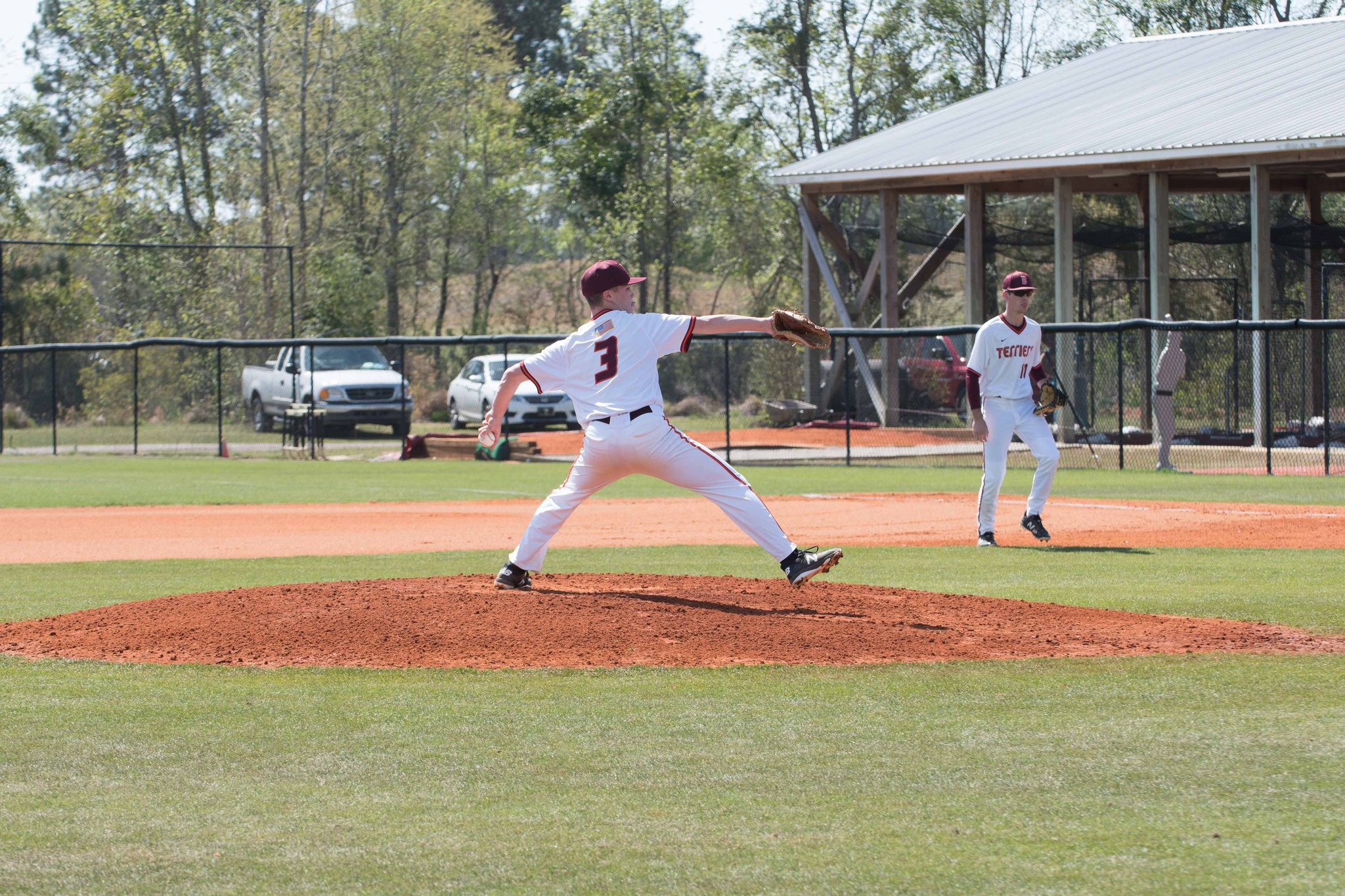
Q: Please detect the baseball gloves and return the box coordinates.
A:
[770,304,833,353]
[1032,379,1068,418]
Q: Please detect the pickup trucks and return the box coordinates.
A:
[240,344,415,436]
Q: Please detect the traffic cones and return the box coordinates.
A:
[220,436,230,457]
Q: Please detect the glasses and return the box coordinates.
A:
[1007,289,1033,297]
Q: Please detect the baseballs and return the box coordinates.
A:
[477,432,496,447]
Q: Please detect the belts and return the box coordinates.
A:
[595,406,652,424]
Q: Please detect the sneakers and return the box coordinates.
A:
[1020,510,1051,542]
[781,546,843,586]
[494,562,531,589]
[977,532,997,547]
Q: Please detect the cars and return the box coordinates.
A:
[446,352,582,431]
[900,330,1058,426]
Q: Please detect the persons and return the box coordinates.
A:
[966,271,1069,547]
[1152,314,1186,471]
[478,260,844,589]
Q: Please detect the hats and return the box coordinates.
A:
[581,260,647,296]
[1002,270,1038,290]
